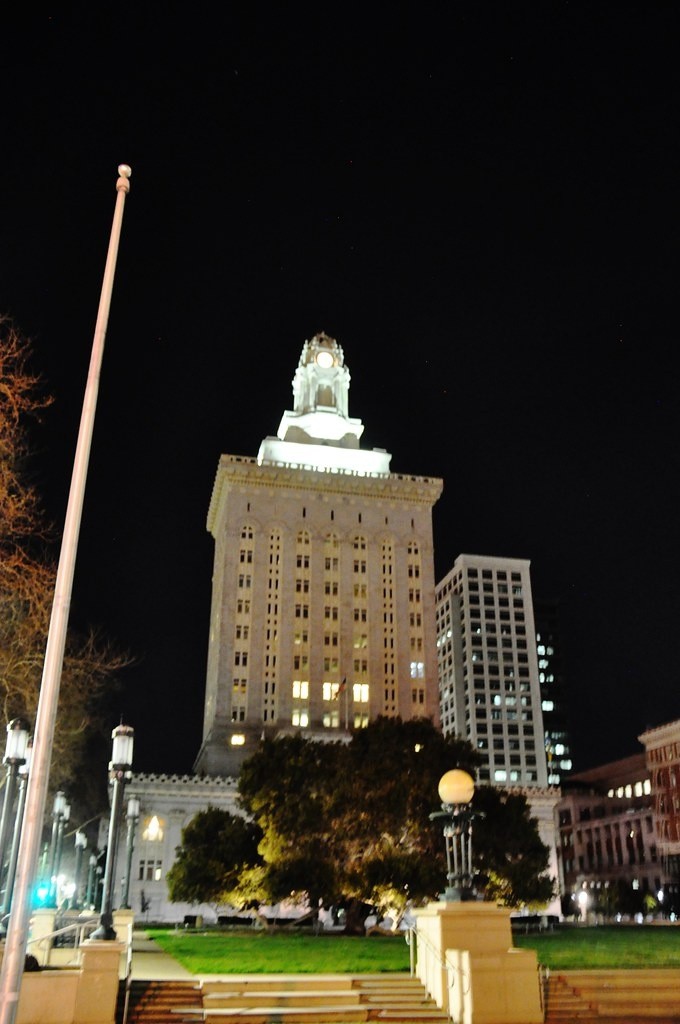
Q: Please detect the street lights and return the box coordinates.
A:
[119,793,143,910]
[0,716,33,865]
[0,734,37,939]
[71,828,90,909]
[90,719,136,941]
[48,802,72,908]
[44,791,66,907]
